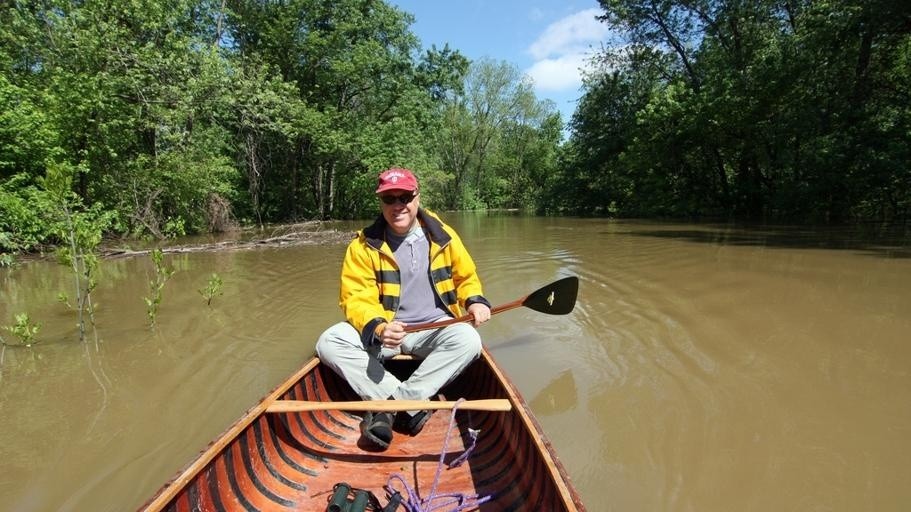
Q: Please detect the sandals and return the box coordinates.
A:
[409,409,432,436]
[362,410,397,449]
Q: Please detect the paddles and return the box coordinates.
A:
[403,276,578,334]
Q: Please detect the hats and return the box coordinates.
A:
[376,168,418,194]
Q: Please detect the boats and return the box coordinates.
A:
[135,309,588,512]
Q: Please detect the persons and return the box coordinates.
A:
[314,169,492,452]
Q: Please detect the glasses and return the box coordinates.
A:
[380,194,418,204]
